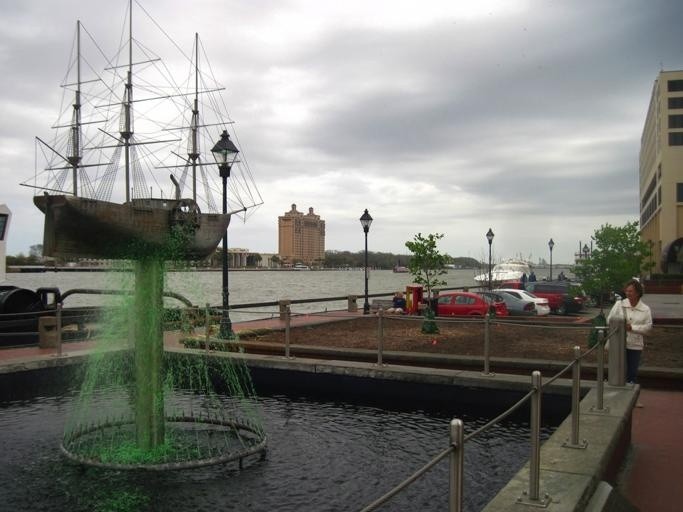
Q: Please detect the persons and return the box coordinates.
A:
[518,272,527,289]
[528,271,535,282]
[385,290,405,315]
[557,271,564,281]
[604,278,653,408]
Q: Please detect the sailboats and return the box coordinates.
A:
[19,2,264,260]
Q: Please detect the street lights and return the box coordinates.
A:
[210,129,241,340]
[358,208,373,314]
[485,227,495,292]
[582,244,590,260]
[548,237,555,282]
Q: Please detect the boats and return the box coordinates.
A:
[472,260,532,291]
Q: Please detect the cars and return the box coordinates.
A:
[426,280,585,319]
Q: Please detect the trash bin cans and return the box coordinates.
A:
[38,316,57,348]
[348,295,356,312]
[280,301,290,321]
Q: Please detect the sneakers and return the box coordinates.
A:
[634,399,643,408]
